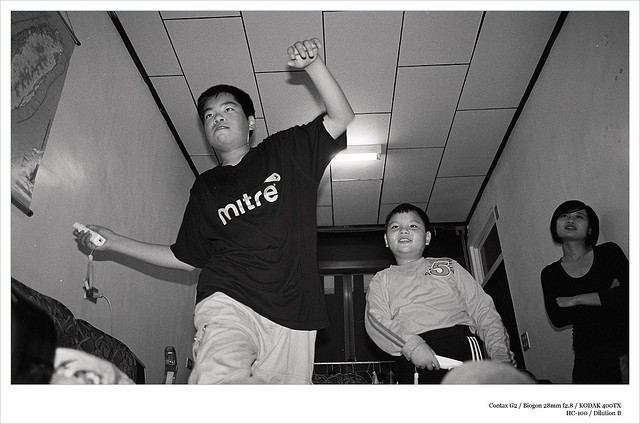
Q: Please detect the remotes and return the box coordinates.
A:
[413,355,463,385]
[73,223,106,291]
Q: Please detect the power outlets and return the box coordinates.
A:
[83,286,99,304]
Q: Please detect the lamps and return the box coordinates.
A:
[332,150,383,164]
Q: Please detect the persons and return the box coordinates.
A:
[365,202,513,385]
[73,39,354,385]
[539,200,629,385]
